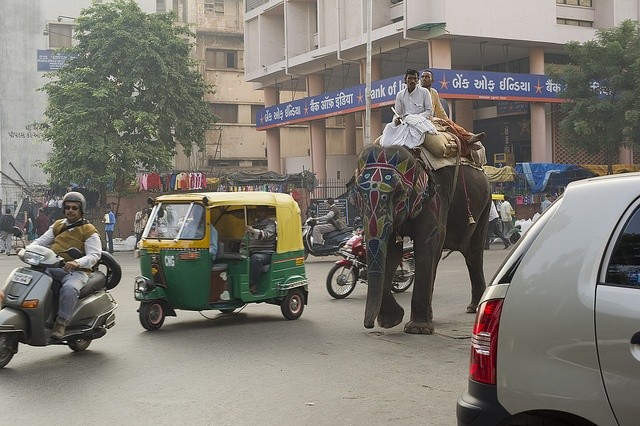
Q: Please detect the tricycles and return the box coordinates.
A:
[134,191,310,332]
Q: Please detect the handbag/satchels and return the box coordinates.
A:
[12,226,23,238]
[333,218,347,231]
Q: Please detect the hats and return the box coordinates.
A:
[327,198,334,205]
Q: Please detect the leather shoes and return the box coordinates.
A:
[251,284,257,294]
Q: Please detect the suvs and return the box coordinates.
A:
[457,171,640,425]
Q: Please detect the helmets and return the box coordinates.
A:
[62,191,86,214]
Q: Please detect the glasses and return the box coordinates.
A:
[65,205,80,210]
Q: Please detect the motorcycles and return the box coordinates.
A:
[302,217,354,261]
[326,217,415,299]
[0,228,122,370]
[489,222,522,245]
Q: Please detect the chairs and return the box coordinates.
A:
[209,241,227,271]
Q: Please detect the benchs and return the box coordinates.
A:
[220,237,276,272]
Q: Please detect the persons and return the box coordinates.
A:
[379,67,439,159]
[102,204,115,254]
[540,194,552,212]
[240,206,277,294]
[0,208,15,255]
[32,208,50,237]
[181,206,219,269]
[134,208,148,249]
[311,197,347,247]
[484,200,512,250]
[501,196,513,236]
[421,69,486,153]
[16,191,104,340]
[24,212,35,243]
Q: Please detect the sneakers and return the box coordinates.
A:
[50,323,66,339]
[0,250,5,253]
[6,252,10,256]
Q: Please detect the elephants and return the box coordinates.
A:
[345,142,493,334]
